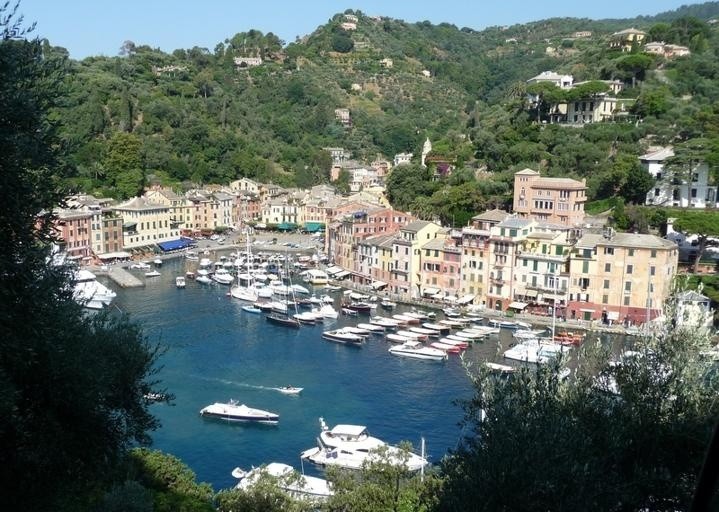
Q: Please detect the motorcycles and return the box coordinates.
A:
[279,383,304,396]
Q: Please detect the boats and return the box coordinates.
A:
[56,271,117,310]
[200,400,280,427]
[386,271,679,402]
[176,232,396,347]
[131,259,162,277]
[232,415,429,508]
